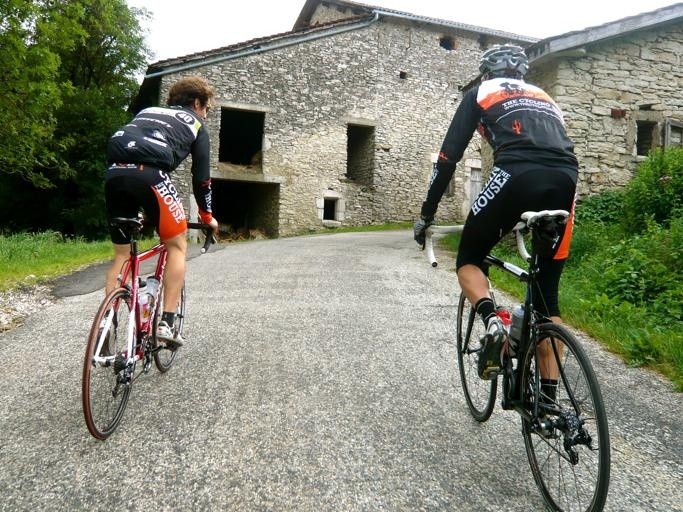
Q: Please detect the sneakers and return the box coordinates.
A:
[92,315,116,367]
[476,315,508,382]
[532,404,560,440]
[154,321,184,348]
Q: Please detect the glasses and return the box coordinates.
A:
[200,100,213,109]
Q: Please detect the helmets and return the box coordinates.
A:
[475,42,529,78]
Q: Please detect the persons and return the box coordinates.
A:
[94,75,219,369]
[413,43,579,438]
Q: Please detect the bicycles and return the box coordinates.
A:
[80,210,217,440]
[410,209,612,511]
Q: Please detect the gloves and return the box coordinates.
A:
[410,216,434,245]
